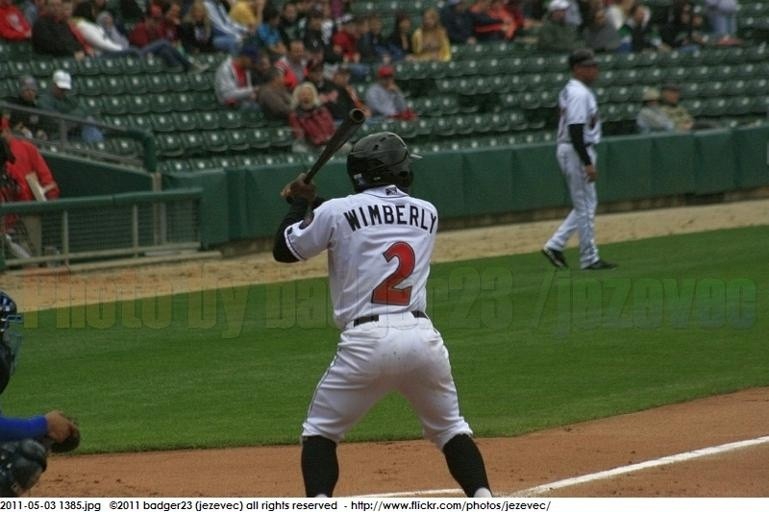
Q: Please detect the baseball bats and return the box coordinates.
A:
[304,109,365,185]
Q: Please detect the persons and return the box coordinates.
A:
[658,86,695,131]
[1,0,764,60]
[0,116,60,261]
[48,69,105,152]
[213,42,413,153]
[10,73,60,140]
[636,86,672,133]
[269,132,498,498]
[0,287,81,498]
[540,51,616,270]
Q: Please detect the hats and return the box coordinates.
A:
[568,48,601,67]
[377,66,393,78]
[306,58,324,72]
[0,293,27,393]
[53,70,71,90]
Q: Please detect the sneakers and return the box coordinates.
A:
[582,258,616,271]
[540,243,570,270]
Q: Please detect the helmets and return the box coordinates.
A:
[345,129,424,193]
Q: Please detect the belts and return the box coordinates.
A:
[353,310,427,326]
